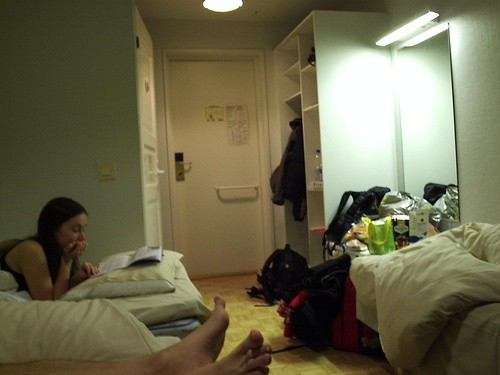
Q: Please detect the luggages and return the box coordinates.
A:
[330,274,384,357]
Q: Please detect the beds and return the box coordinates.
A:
[0,248,213,366]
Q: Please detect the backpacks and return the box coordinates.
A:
[245,243,309,306]
[323,186,391,243]
[424,183,456,204]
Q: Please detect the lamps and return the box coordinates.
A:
[374,7,441,47]
[202,0,243,13]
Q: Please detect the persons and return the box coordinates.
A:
[0,197,97,300]
[0,296,272,375]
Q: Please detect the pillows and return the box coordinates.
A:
[62,249,184,295]
[107,264,213,325]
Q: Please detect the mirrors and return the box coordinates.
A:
[397,22,460,221]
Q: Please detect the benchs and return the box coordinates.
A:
[349,222,500,375]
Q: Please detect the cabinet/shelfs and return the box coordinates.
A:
[272,11,399,268]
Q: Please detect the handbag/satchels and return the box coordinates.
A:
[433,186,459,221]
[378,191,441,228]
